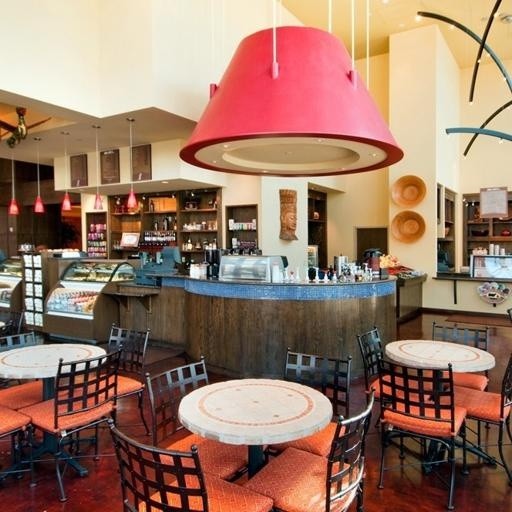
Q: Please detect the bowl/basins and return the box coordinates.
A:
[444,225,448,236]
[471,228,488,236]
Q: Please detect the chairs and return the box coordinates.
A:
[355,322,511,510]
[1,322,151,504]
[106,346,377,511]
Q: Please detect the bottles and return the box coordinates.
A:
[154,215,177,231]
[145,231,176,245]
[501,226,510,236]
[339,262,374,282]
[150,200,155,212]
[182,235,217,251]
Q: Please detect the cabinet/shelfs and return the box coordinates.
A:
[437,183,455,242]
[109,193,142,252]
[42,261,137,342]
[467,200,512,243]
[0,274,23,330]
[140,192,178,246]
[178,187,222,254]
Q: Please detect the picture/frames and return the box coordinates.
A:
[70,154,88,187]
[100,148,120,184]
[132,143,152,181]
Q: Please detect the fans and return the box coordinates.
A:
[0,107,53,148]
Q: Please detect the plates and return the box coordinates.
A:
[390,211,425,243]
[391,175,427,207]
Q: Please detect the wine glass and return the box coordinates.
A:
[281,265,338,283]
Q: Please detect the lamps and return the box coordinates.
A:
[125,116,139,210]
[8,143,21,216]
[176,0,406,178]
[59,130,73,211]
[31,135,46,213]
[90,123,105,211]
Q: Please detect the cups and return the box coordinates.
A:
[489,244,505,256]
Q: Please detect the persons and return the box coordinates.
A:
[279,203,298,240]
